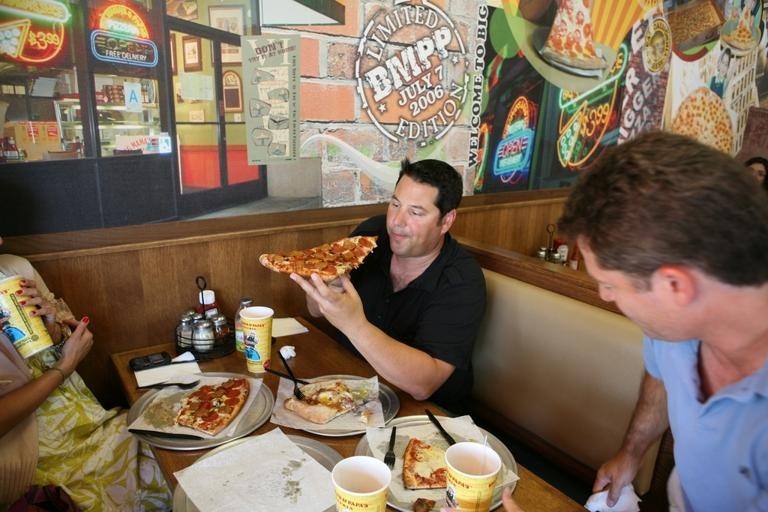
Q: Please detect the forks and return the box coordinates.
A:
[383,424,396,466]
[135,379,201,391]
[276,350,307,399]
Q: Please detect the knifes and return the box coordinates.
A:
[424,407,455,446]
[264,367,311,385]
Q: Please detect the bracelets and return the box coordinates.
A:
[49,367,65,385]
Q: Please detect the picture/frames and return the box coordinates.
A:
[182,36,202,71]
[208,5,247,67]
[169,33,178,76]
[222,70,242,113]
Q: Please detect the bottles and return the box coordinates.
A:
[236,300,253,353]
[569,241,581,271]
[196,291,220,318]
[177,309,227,352]
[536,239,567,266]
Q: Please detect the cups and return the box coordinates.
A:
[330,455,391,512]
[444,443,501,512]
[241,306,274,373]
[0,274,55,360]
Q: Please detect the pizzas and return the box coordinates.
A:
[285,379,356,425]
[541,0,607,69]
[177,379,249,435]
[672,87,733,156]
[403,438,447,489]
[258,235,379,282]
[721,26,757,49]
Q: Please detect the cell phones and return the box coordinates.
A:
[129,351,171,372]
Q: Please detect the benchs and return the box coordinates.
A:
[455,235,662,509]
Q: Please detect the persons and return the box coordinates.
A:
[555,129,768,512]
[744,156,768,185]
[0,235,174,511]
[290,159,487,418]
